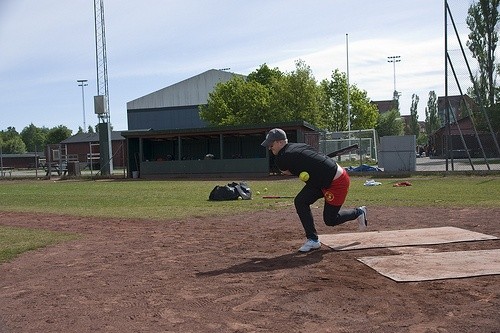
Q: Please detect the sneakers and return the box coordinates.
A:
[298,239,321,251]
[357,205,368,230]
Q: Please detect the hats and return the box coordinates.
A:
[261,128,286,148]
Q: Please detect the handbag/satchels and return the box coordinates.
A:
[209,182,243,200]
[235,182,252,199]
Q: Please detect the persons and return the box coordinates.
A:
[261,127,367,252]
[416,144,438,158]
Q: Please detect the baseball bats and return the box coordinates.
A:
[327,143,359,159]
[263,196,295,199]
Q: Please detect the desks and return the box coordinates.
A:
[0,167,14,177]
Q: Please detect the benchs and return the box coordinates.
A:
[42,165,67,176]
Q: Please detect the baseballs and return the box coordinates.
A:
[263,187,268,192]
[237,196,242,200]
[299,172,309,181]
[256,191,261,195]
